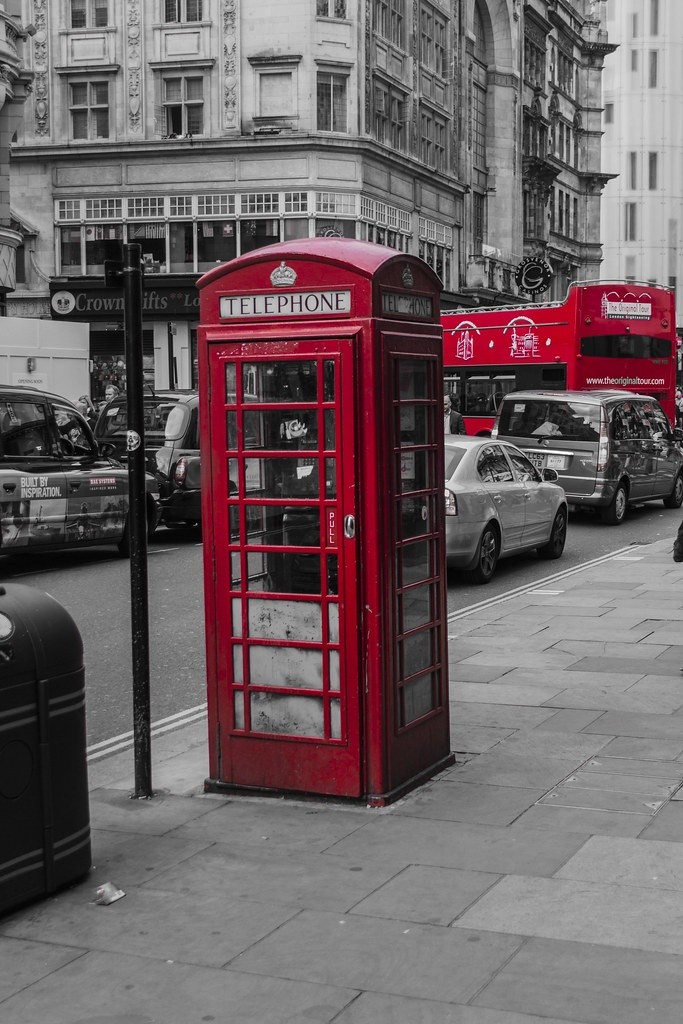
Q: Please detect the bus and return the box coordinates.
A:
[440,279,677,437]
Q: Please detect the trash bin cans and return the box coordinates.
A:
[0,581,91,929]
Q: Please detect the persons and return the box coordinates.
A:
[675,388,683,430]
[448,381,504,415]
[443,394,467,435]
[55,358,126,438]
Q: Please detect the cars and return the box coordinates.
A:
[444,434,569,583]
[0,384,161,559]
[92,391,266,533]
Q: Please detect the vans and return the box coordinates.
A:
[490,389,682,525]
